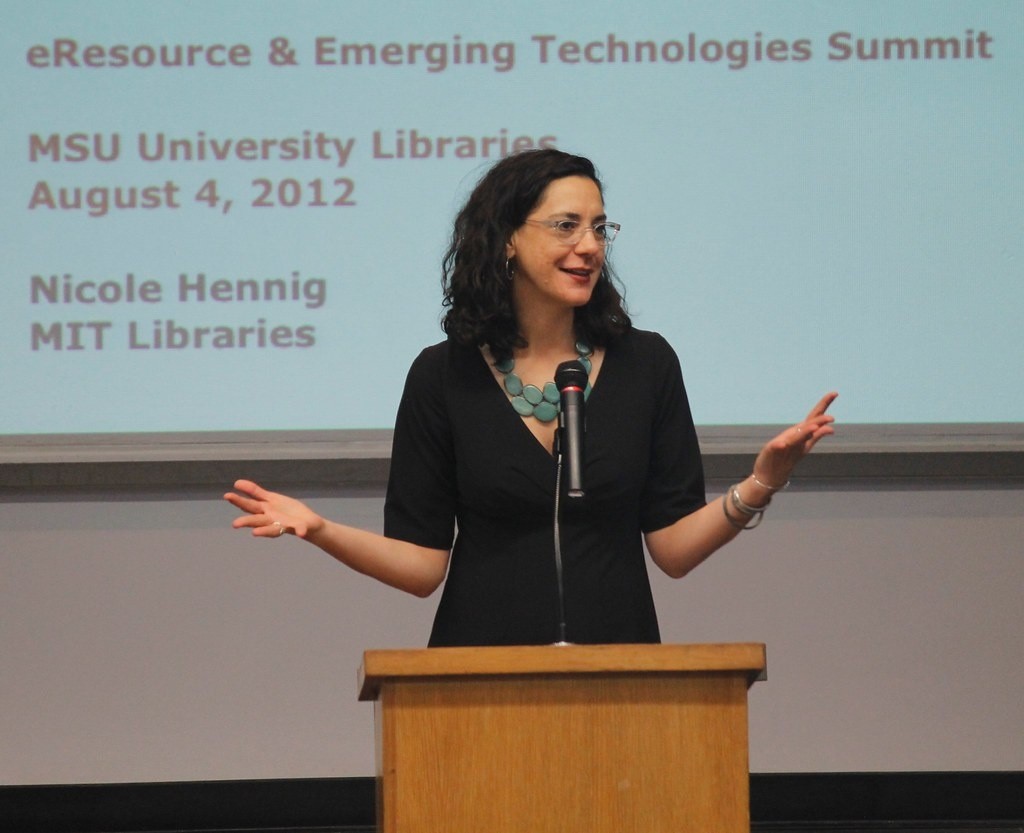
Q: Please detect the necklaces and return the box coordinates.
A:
[494,340,592,422]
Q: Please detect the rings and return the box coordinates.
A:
[272,521,283,535]
[796,427,801,432]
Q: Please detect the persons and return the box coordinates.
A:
[225,148,838,648]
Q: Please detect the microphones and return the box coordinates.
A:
[554,360,588,497]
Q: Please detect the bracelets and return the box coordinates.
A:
[722,474,791,531]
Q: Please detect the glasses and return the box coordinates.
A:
[523,219,621,246]
